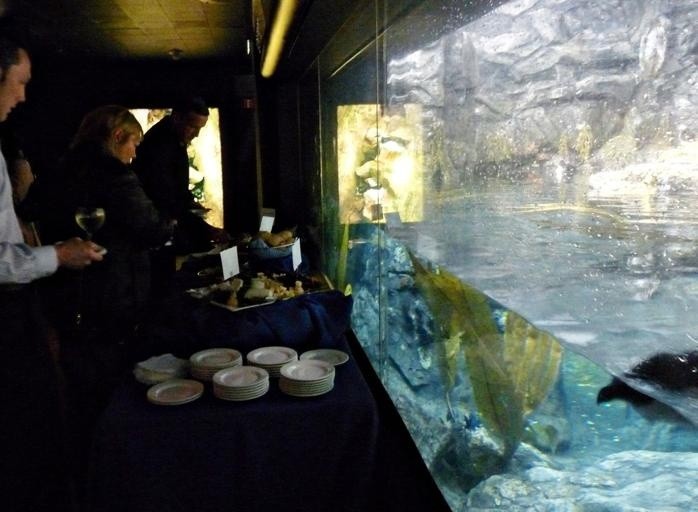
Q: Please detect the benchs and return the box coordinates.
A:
[72,240,380,512]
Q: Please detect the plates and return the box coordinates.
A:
[145,346,337,408]
[299,348,351,369]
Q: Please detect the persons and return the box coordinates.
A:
[0,40,232,511]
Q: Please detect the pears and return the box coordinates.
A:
[257,230,293,247]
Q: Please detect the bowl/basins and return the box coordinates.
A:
[248,238,295,259]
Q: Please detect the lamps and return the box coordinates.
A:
[168,48,183,62]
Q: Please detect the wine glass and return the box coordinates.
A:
[73,204,106,260]
